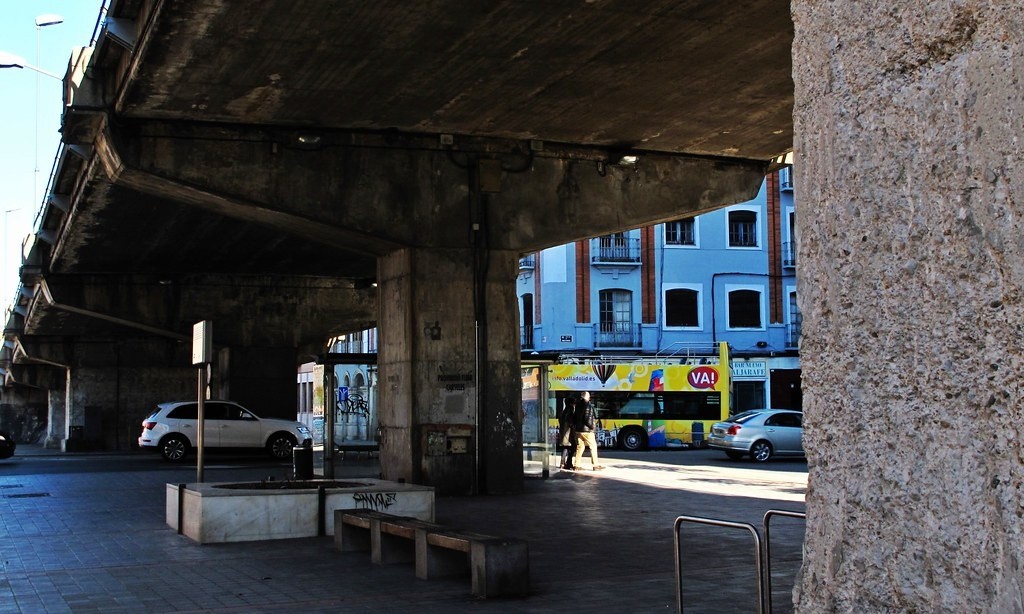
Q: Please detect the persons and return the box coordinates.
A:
[557,397,574,469]
[572,390,606,471]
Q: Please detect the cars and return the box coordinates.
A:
[708,407,806,462]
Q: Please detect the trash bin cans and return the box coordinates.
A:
[292,444,314,480]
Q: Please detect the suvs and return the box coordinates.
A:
[137,401,313,464]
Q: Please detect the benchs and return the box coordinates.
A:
[334,507,530,600]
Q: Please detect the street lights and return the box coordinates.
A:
[0,50,62,82]
[33,12,62,226]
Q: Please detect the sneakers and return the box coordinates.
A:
[573,467,585,471]
[593,466,605,470]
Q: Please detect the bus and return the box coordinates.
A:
[523,339,733,453]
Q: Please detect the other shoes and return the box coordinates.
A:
[560,464,573,469]
